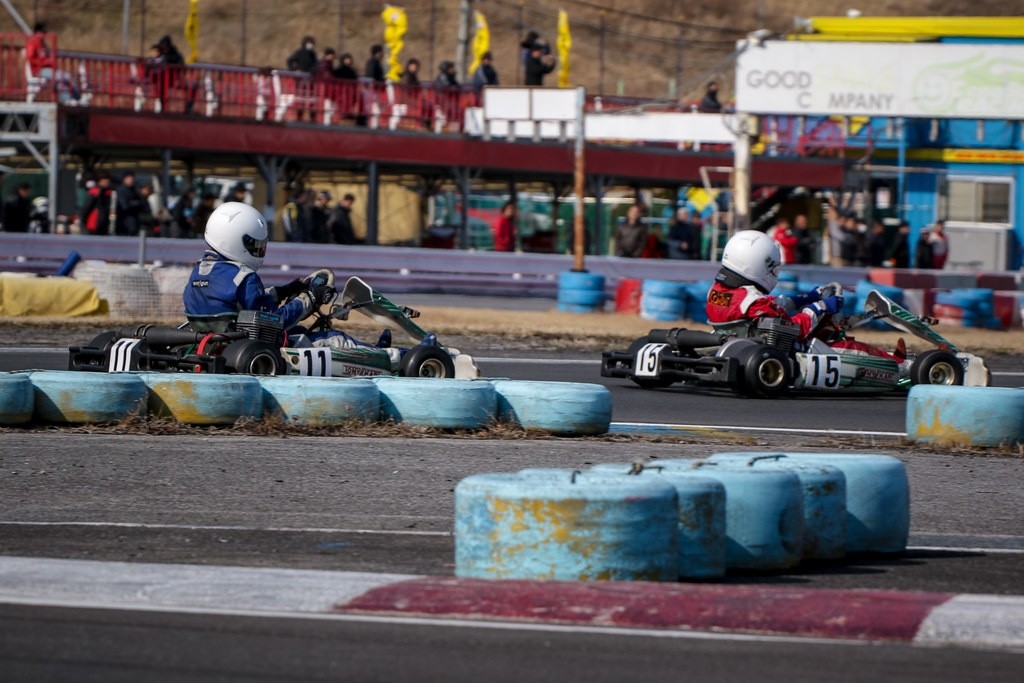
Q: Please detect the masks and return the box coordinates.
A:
[305,43,314,51]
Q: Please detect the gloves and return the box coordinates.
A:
[280,276,309,297]
[789,286,822,309]
[808,295,844,316]
[302,284,336,307]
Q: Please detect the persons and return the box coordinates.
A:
[526,48,555,85]
[435,60,462,118]
[0,171,357,243]
[399,58,433,130]
[131,32,184,111]
[184,202,436,372]
[494,201,516,252]
[317,46,357,119]
[474,51,499,94]
[699,80,722,113]
[615,206,647,258]
[706,230,948,378]
[25,21,81,101]
[667,207,948,269]
[356,44,385,126]
[288,36,317,121]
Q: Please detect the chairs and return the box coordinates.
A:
[707,317,752,339]
[183,311,240,332]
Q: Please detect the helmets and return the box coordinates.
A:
[715,230,781,295]
[203,202,268,271]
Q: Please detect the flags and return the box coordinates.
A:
[183,0,200,64]
[470,12,490,74]
[556,10,572,87]
[381,5,408,83]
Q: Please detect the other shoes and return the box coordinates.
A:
[893,338,906,359]
[939,343,951,353]
[421,333,436,346]
[375,329,392,349]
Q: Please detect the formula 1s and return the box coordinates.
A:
[598,280,991,402]
[65,264,483,382]
